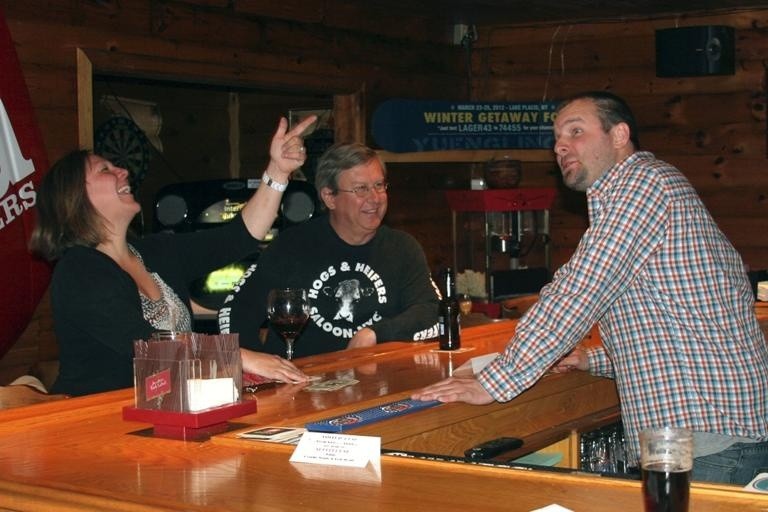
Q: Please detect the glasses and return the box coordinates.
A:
[333,182,389,197]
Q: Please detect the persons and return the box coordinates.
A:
[409,90,768,487]
[27,114,319,396]
[217,141,444,359]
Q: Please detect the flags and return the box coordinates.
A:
[0,7,53,359]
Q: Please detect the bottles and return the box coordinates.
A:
[581,423,640,476]
[437,267,461,350]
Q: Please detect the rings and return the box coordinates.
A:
[300,147,303,152]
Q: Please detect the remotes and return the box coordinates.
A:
[464,437,524,460]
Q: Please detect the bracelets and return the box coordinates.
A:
[262,170,288,192]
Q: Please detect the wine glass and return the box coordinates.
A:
[268,286,309,360]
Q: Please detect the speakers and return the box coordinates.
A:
[655,24,735,78]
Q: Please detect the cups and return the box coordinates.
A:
[639,427,693,511]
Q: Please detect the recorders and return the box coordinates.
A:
[152,178,320,244]
